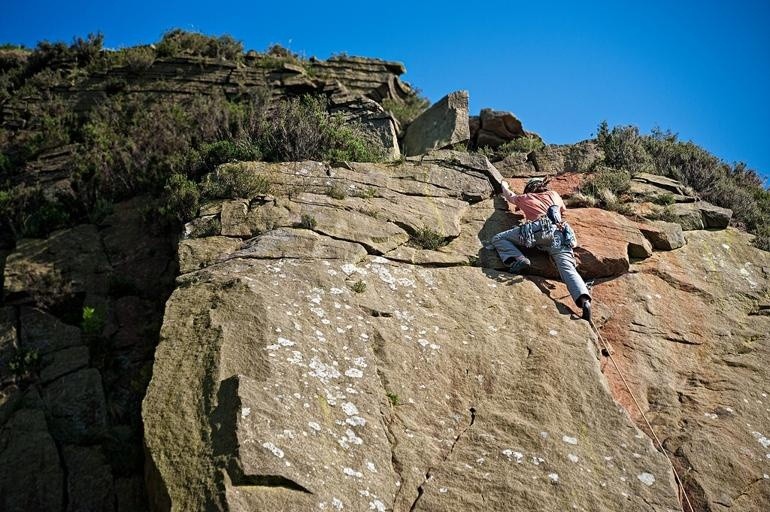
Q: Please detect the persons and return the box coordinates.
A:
[492,178,592,321]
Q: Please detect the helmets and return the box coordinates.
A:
[524,178,547,192]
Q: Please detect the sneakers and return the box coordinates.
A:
[507,258,531,274]
[583,302,591,321]
